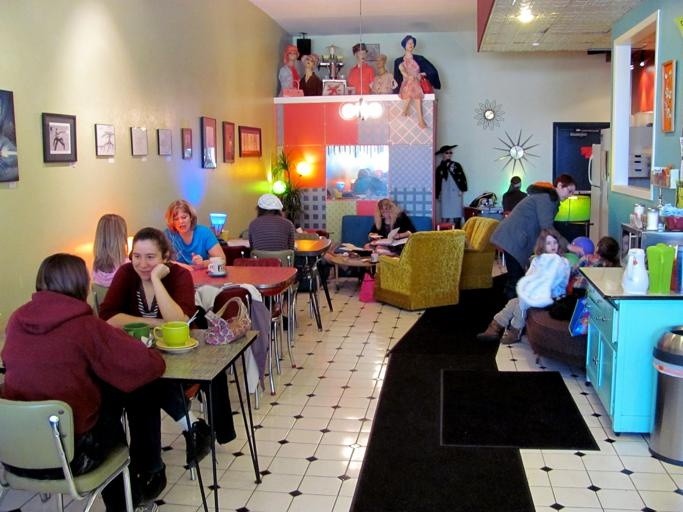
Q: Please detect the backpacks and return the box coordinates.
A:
[470,192,496,208]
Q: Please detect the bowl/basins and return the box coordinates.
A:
[660,215,683,232]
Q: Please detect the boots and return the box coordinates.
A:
[502,326,521,344]
[477,320,503,339]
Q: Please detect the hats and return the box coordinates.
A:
[435,144,457,155]
[258,194,284,210]
[301,53,320,68]
[284,45,300,64]
[353,43,368,56]
[401,36,416,49]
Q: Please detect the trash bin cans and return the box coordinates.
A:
[648,326,683,467]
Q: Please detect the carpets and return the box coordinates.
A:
[439,367,599,451]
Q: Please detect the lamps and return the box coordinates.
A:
[209,214,228,254]
[554,195,591,237]
[338,0,384,122]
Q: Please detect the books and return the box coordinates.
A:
[375,227,409,248]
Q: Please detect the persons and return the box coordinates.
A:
[160,199,226,271]
[276,34,439,127]
[475,174,619,344]
[364,199,417,255]
[434,145,466,230]
[248,193,296,315]
[2,252,164,512]
[100,228,214,499]
[91,213,133,287]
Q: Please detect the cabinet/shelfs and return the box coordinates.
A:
[273,94,438,279]
[585,266,683,438]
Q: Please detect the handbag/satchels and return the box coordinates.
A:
[283,88,304,97]
[421,79,433,94]
[359,273,375,302]
[549,293,588,337]
[204,303,251,345]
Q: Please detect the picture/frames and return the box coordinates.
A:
[0,90,261,184]
[661,58,674,133]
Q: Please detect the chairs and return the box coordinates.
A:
[372,229,464,310]
[457,215,500,291]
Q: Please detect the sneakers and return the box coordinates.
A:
[133,463,168,512]
[182,418,216,465]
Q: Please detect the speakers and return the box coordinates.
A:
[297,39,311,61]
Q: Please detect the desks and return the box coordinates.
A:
[319,246,399,292]
[465,207,503,222]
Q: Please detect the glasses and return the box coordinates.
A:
[566,185,574,196]
[443,152,453,155]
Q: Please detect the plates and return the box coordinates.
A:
[155,338,199,354]
[370,261,379,264]
[207,272,228,277]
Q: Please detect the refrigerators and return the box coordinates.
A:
[588,144,601,256]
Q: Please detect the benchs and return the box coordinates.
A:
[341,215,432,284]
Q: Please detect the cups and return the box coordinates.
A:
[121,323,150,342]
[370,253,378,262]
[220,230,229,242]
[152,321,189,346]
[630,203,658,232]
[208,257,226,275]
[646,243,683,295]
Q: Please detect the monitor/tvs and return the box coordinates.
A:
[553,122,610,190]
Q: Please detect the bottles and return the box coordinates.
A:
[621,248,648,295]
